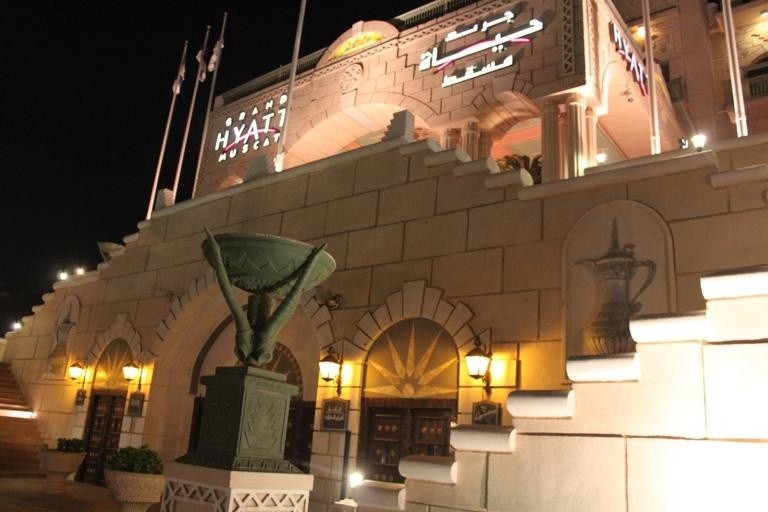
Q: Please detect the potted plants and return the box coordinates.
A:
[103,446,165,512]
[38,436,87,496]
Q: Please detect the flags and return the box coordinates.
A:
[195,28,208,85]
[172,43,188,97]
[207,25,225,72]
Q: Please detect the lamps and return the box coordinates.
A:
[121,355,139,381]
[318,346,341,382]
[68,356,83,380]
[465,336,491,379]
